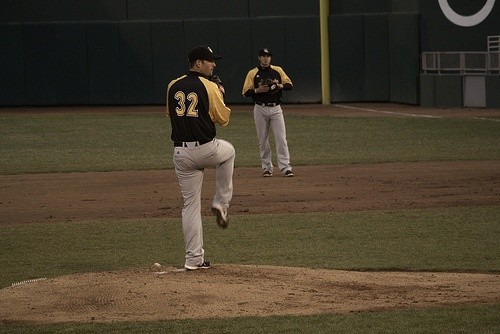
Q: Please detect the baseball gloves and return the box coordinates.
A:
[260,79,281,94]
[208,75,222,84]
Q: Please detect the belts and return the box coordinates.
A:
[174,141,210,148]
[256,101,280,107]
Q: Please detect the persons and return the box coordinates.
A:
[167,45,235,271]
[242,48,294,176]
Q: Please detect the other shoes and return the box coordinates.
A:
[262,171,272,176]
[185,260,211,270]
[212,204,229,229]
[285,170,294,177]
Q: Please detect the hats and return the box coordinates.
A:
[258,48,273,57]
[188,47,223,62]
